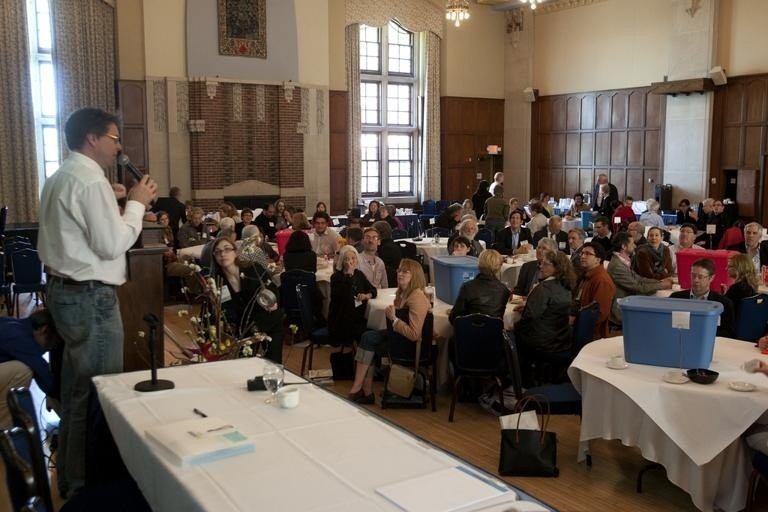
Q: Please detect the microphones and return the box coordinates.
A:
[117,153,159,204]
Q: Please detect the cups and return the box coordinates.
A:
[277,386,300,409]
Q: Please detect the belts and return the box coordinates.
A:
[46,275,101,287]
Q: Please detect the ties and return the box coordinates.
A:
[513,232,518,249]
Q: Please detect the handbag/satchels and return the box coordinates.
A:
[478,386,560,478]
[382,363,426,409]
[330,350,357,381]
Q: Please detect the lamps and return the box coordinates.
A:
[444,0,470,28]
[488,145,498,184]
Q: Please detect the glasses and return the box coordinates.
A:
[213,248,237,257]
[691,273,715,280]
[543,262,555,266]
[91,130,120,143]
[396,269,412,274]
[580,251,595,257]
[364,236,379,240]
[681,230,695,233]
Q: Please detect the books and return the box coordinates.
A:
[144,415,255,468]
[498,410,542,432]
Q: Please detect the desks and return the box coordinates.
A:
[91,356,550,512]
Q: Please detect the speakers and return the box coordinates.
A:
[524,87,538,103]
[655,184,673,209]
[709,67,727,86]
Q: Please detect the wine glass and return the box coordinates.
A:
[263,364,286,403]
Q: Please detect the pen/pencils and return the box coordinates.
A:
[194,408,207,418]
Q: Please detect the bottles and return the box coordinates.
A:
[424,282,436,308]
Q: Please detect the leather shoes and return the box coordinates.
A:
[361,393,376,404]
[347,388,367,402]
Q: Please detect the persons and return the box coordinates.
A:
[446,250,510,328]
[347,259,433,402]
[577,242,616,341]
[428,171,638,294]
[745,361,768,511]
[606,233,672,331]
[754,335,768,354]
[37,105,158,510]
[641,194,768,332]
[0,310,60,432]
[513,250,576,389]
[109,185,404,363]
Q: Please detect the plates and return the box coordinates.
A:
[605,357,761,393]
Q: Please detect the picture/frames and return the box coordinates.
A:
[217,0,267,59]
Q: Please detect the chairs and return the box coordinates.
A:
[0,206,48,320]
[0,427,39,512]
[7,386,136,512]
[21,496,49,512]
